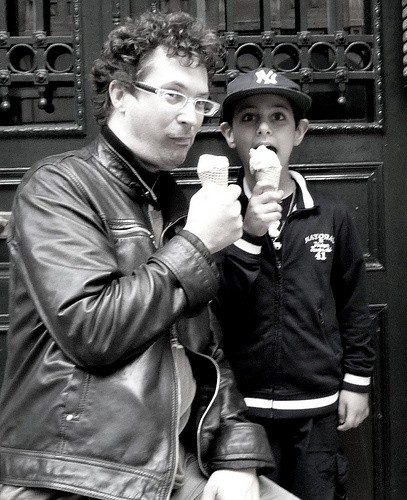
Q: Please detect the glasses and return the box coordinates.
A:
[127,81,220,117]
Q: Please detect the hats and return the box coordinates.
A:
[222,68,311,122]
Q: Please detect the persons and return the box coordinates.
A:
[0,7,301,500]
[214,66,378,500]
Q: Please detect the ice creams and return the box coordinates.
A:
[197,154,229,188]
[249,144,283,237]
